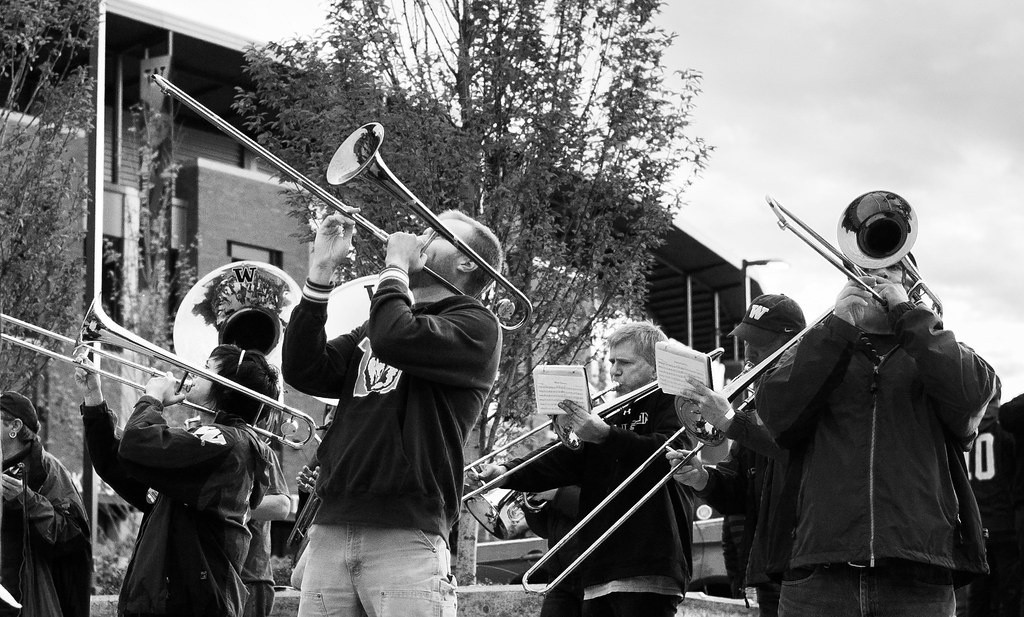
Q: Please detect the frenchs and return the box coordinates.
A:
[173,260,303,377]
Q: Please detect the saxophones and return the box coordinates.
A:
[286,463,324,550]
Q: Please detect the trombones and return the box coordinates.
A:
[766,191,943,328]
[1,298,316,451]
[467,484,529,540]
[460,350,726,503]
[522,305,838,597]
[152,74,532,334]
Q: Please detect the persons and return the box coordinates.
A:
[666,294,806,617]
[244,442,291,617]
[469,321,696,617]
[953,394,1024,617]
[73,343,280,617]
[282,207,504,617]
[755,249,1002,617]
[0,391,93,617]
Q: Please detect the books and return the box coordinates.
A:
[654,342,713,399]
[532,361,592,415]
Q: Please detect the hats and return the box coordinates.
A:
[0,391,39,434]
[726,293,807,347]
